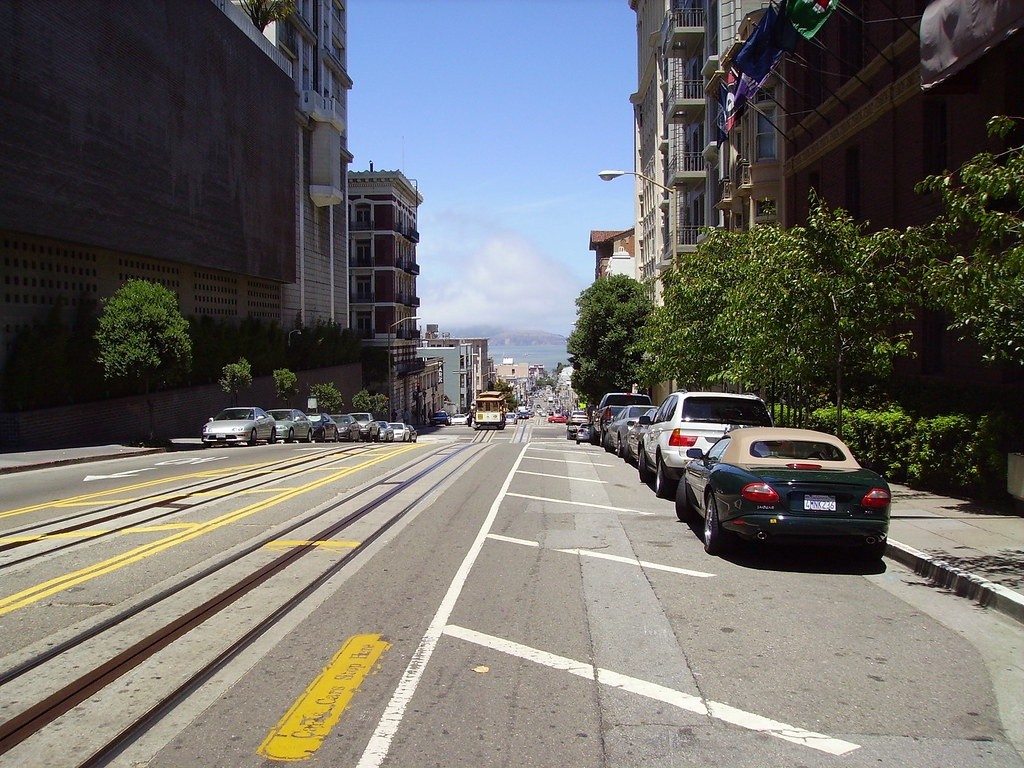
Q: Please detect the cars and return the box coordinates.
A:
[548,416,568,423]
[676,426,892,558]
[430,412,451,426]
[519,412,529,419]
[506,413,519,425]
[266,409,417,443]
[567,389,659,461]
[202,407,277,447]
[451,414,468,425]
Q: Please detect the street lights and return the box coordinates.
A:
[388,317,421,423]
[288,330,301,346]
[598,171,677,272]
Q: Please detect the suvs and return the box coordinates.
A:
[637,388,776,497]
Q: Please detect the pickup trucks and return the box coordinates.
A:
[590,393,653,445]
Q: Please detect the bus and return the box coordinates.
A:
[471,391,506,430]
